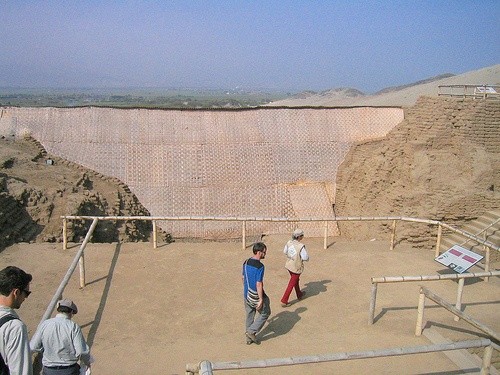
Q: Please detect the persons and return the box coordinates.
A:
[0,265,34,375]
[28,298,95,375]
[241,242,271,345]
[280,228,310,307]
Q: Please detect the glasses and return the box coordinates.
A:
[20,290,31,297]
[262,251,267,254]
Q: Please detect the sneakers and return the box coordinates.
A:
[245,330,261,345]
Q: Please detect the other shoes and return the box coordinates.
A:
[281,301,293,307]
[297,290,307,299]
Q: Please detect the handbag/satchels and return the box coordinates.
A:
[245,288,264,308]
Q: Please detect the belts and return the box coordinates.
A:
[44,362,78,370]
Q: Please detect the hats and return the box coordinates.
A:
[56,299,78,314]
[292,229,305,238]
